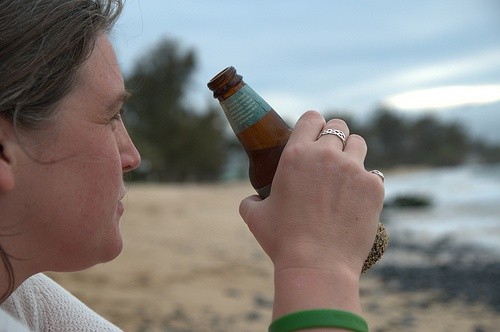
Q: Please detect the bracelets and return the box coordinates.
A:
[268,308,369,332]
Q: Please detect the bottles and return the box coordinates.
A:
[208,66,389,274]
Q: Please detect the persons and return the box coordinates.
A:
[0,0,385,332]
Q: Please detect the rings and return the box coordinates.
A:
[317,128,346,146]
[369,170,384,180]
